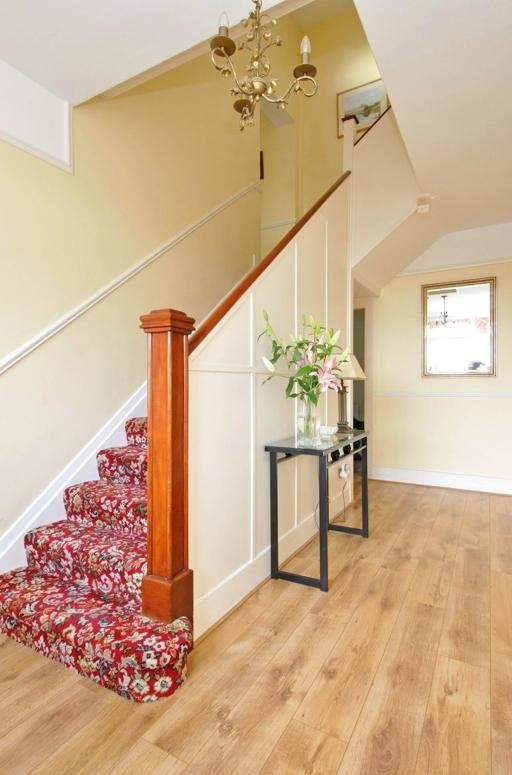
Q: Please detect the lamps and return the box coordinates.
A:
[330,354,366,433]
[211,0,318,131]
[435,295,449,325]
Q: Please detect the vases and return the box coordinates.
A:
[296,391,321,446]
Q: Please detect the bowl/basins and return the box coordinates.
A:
[320,426,338,435]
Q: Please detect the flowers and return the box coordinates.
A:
[256,309,349,407]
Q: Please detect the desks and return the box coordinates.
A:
[265,430,368,592]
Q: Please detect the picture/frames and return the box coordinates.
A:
[337,79,390,138]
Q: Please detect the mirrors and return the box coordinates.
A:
[421,277,495,377]
